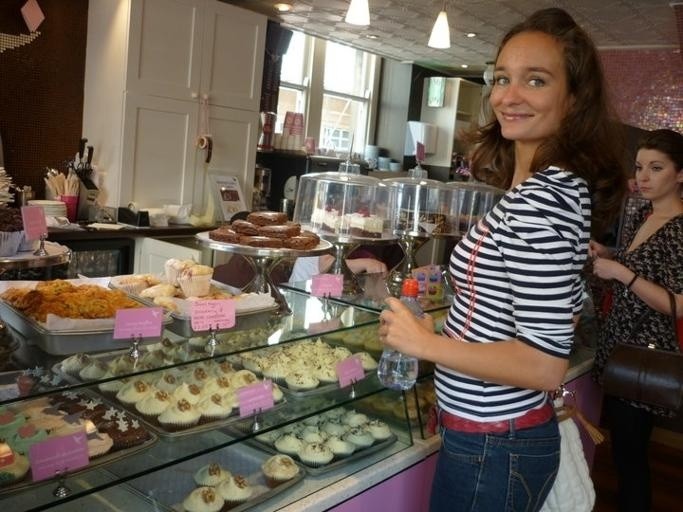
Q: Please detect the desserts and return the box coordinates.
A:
[0,258,437,512]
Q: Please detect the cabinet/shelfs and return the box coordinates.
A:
[418,76,484,170]
[78,0,271,111]
[78,99,261,231]
[0,268,607,512]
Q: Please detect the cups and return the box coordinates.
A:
[58,193,78,224]
[252,166,272,212]
[272,111,315,155]
[17,191,36,207]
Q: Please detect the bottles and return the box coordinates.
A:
[377,276,425,391]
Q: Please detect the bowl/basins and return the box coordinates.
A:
[364,145,400,173]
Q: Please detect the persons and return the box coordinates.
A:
[588,125,682,512]
[374,5,630,512]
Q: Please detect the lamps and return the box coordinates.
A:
[343,0,371,27]
[426,0,451,49]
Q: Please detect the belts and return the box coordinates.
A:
[427,404,554,434]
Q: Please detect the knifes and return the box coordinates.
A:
[77,137,95,178]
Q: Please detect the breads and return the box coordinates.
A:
[208,210,320,250]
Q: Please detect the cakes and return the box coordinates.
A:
[309,203,480,239]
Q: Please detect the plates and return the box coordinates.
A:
[25,199,66,221]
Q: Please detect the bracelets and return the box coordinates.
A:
[621,273,640,298]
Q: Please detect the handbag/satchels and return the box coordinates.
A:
[597,343,682,413]
[539,398,596,512]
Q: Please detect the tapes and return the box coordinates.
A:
[198,136,212,163]
